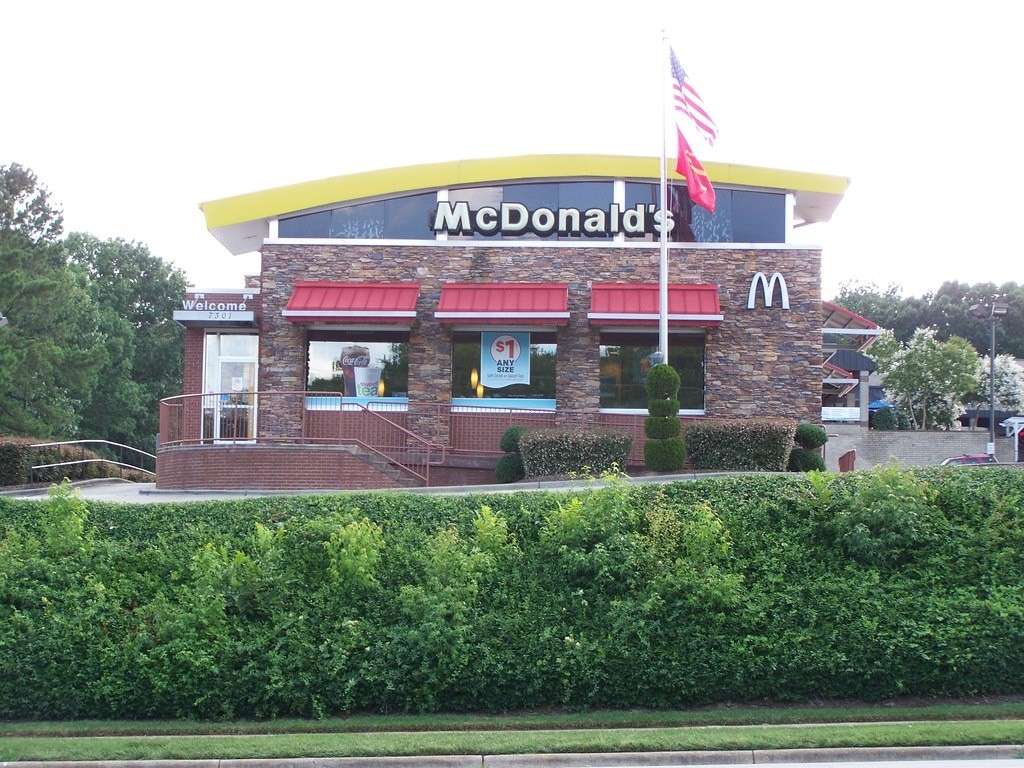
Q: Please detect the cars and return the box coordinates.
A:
[940,453,1000,466]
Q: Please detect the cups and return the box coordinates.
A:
[354,367,383,398]
[341,348,371,397]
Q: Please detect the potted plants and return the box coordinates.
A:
[824,395,844,407]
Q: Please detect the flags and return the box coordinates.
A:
[674,123,717,214]
[669,45,720,147]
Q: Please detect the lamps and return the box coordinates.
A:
[378,378,385,396]
[470,368,478,390]
[476,382,484,398]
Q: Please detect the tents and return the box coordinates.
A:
[869,400,896,413]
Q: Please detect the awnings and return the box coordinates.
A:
[823,362,858,397]
[587,283,724,326]
[282,282,420,322]
[433,283,573,325]
[823,301,882,352]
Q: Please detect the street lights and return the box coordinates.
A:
[968,301,1009,463]
